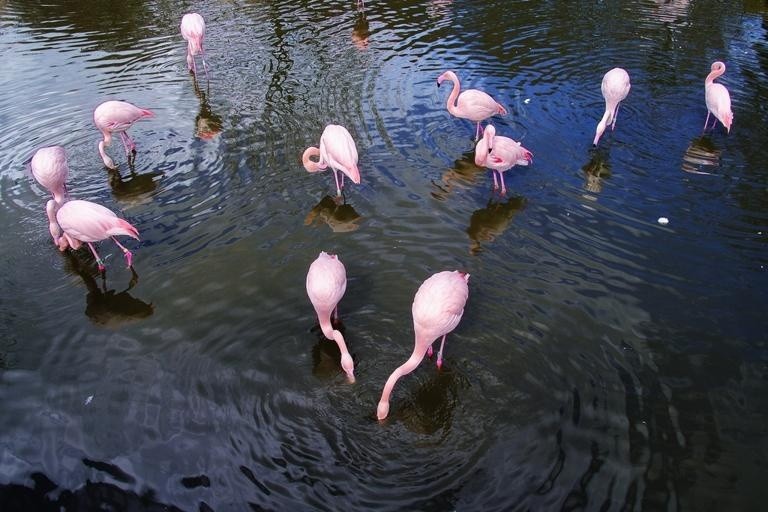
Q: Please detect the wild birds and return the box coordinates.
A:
[301,122,362,201]
[472,123,534,199]
[304,249,357,386]
[375,268,472,424]
[54,198,142,274]
[92,99,153,171]
[435,68,508,144]
[29,145,69,247]
[178,12,212,87]
[702,60,735,149]
[591,67,632,152]
